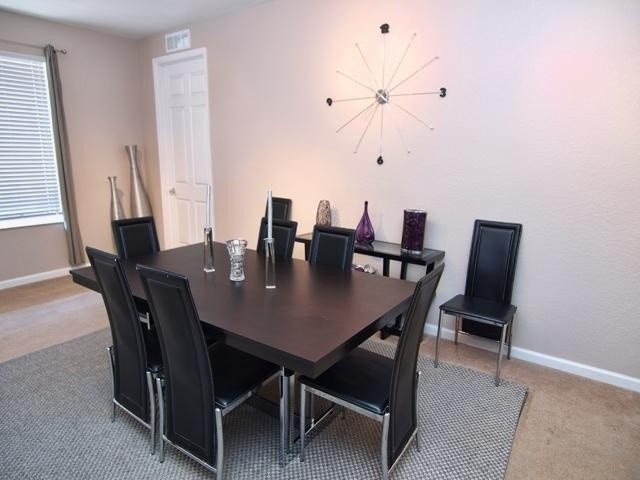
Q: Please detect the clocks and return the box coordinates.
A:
[327,21,450,168]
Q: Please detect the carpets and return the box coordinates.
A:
[0,324,529,480]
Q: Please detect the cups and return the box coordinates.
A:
[226,239,248,281]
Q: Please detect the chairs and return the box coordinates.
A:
[135,261,290,480]
[110,216,163,259]
[299,261,445,478]
[85,245,225,457]
[307,224,358,270]
[434,217,523,387]
[257,217,299,260]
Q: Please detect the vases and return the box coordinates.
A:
[107,144,153,220]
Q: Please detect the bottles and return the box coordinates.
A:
[355,201,375,244]
[265,237,277,289]
[109,145,153,220]
[204,227,216,273]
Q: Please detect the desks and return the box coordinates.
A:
[69,241,419,468]
[296,228,445,287]
